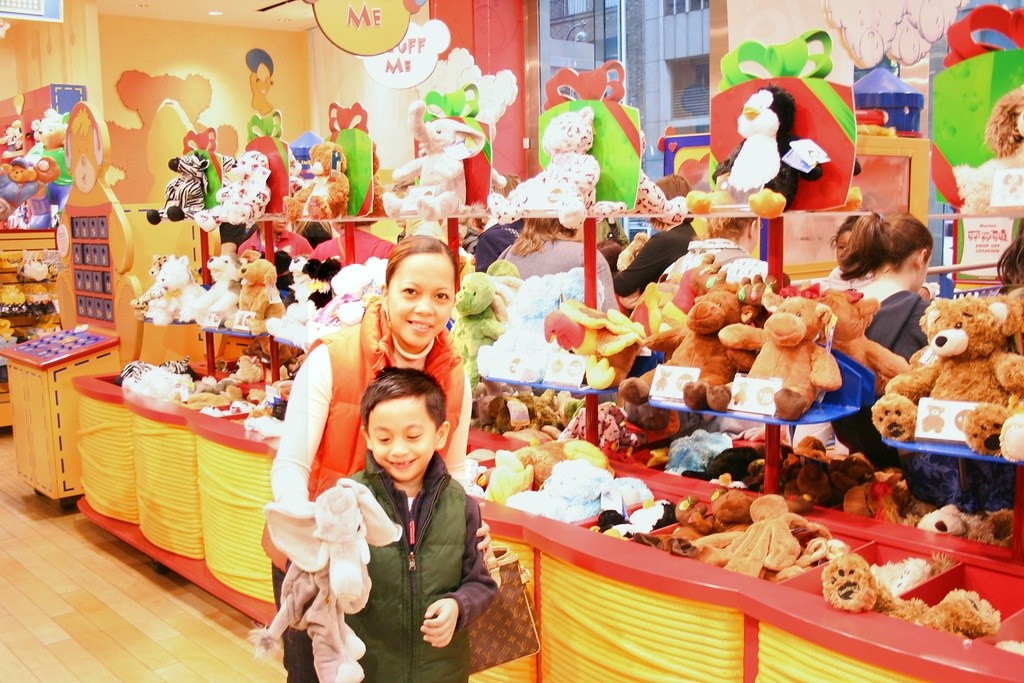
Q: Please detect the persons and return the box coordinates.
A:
[821,216,934,308]
[839,212,934,364]
[344,366,503,683]
[219,217,791,313]
[997,218,1023,288]
[261,235,491,683]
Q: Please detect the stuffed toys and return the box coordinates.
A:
[282,142,350,223]
[487,108,627,229]
[368,99,486,220]
[247,478,404,683]
[628,85,861,218]
[953,83,1024,214]
[0,249,61,379]
[114,248,1024,639]
[194,151,272,233]
[147,152,209,225]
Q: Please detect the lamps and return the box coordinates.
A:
[574,26,591,42]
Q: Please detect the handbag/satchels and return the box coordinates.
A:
[467,545,541,675]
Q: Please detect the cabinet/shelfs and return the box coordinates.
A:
[0,228,62,428]
[71,207,1024,683]
